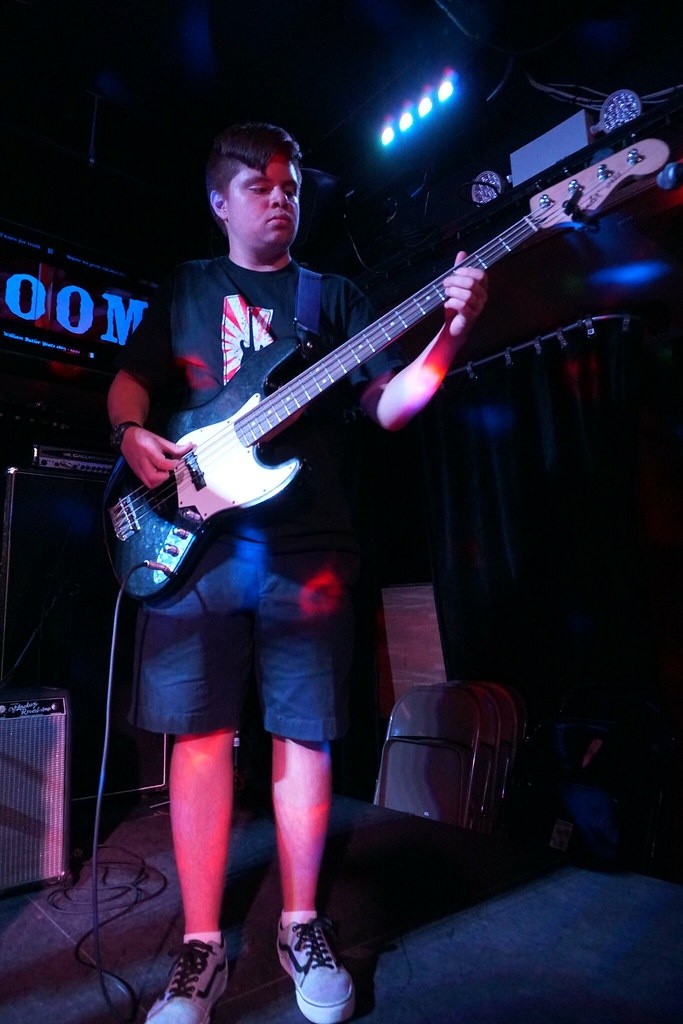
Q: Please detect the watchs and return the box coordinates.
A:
[109,421,142,456]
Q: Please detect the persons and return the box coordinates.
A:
[107,123,487,1024]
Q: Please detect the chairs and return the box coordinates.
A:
[373,680,529,835]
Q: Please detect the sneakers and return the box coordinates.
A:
[146,935,229,1024]
[275,910,357,1024]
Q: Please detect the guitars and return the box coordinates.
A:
[103,136,672,606]
